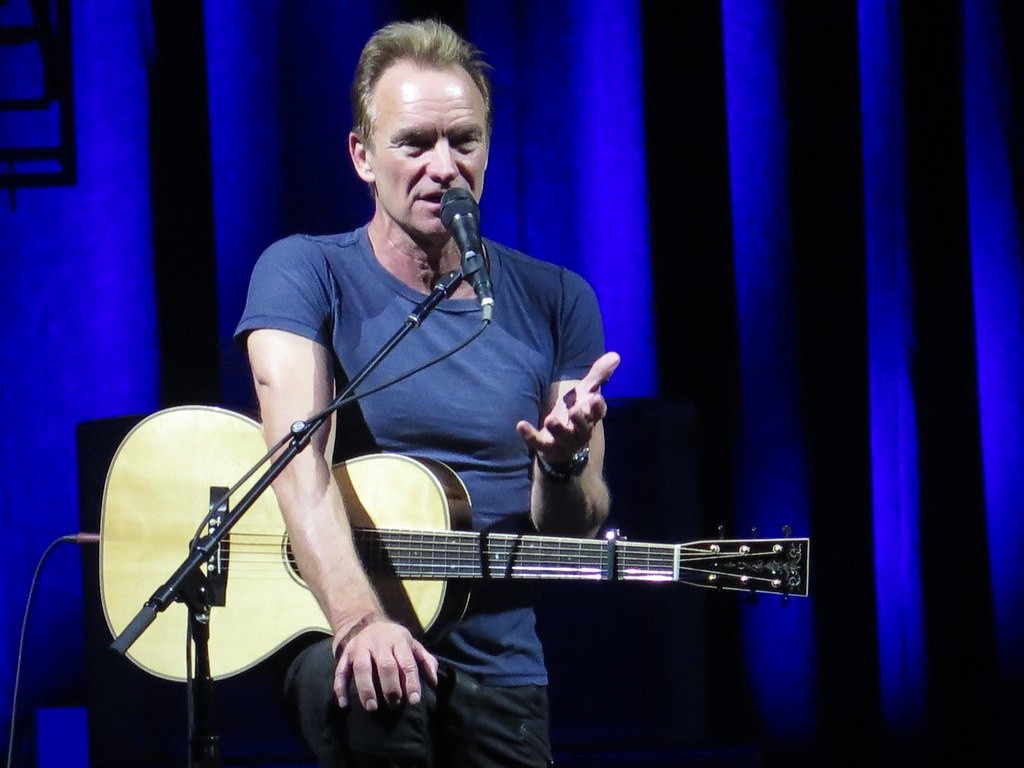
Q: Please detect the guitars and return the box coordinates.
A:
[99,403,809,688]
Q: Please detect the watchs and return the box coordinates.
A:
[538,444,589,482]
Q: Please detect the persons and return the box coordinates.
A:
[234,19,622,768]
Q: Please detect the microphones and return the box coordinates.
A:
[439,187,496,324]
